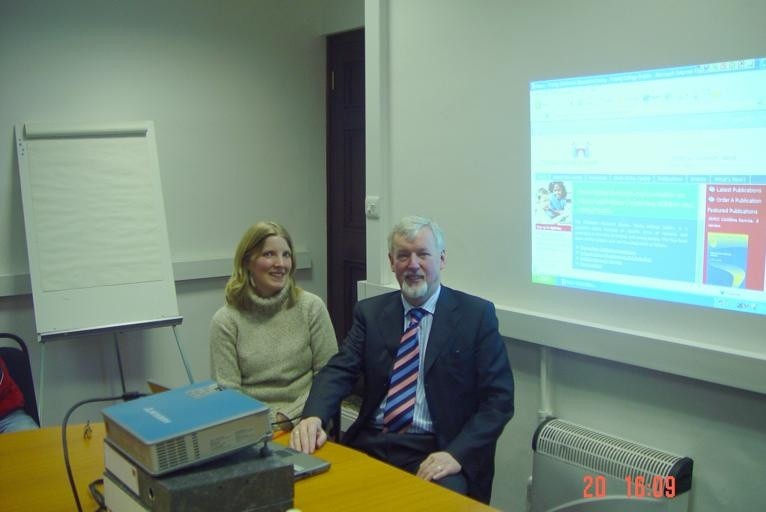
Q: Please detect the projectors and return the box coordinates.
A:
[101,379,272,478]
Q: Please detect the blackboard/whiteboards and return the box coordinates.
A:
[15,121,183,343]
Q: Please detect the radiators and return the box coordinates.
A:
[527,416,692,512]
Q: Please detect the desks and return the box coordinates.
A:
[0,422,496,511]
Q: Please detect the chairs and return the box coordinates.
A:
[0,332,40,428]
[546,495,662,512]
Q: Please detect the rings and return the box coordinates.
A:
[437,463,442,469]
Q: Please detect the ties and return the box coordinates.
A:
[381,309,429,437]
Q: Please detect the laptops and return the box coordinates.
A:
[149,382,331,485]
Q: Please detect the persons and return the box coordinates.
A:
[289,216,515,507]
[205,218,339,435]
